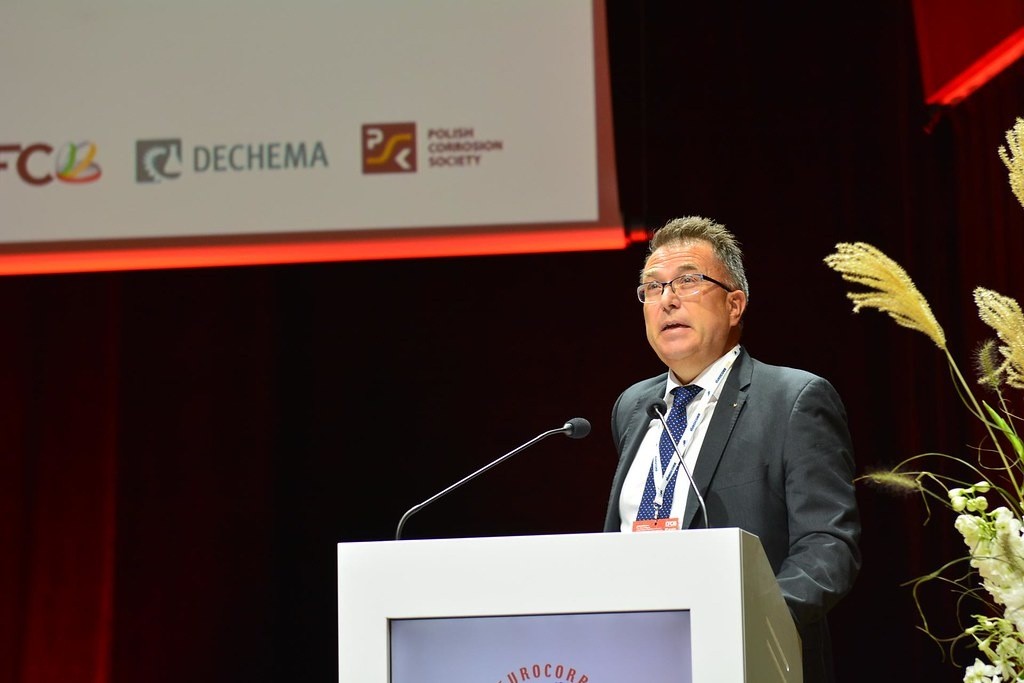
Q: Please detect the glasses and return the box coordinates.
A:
[636,273,731,304]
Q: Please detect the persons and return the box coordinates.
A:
[604,217,864,683]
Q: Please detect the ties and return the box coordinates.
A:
[637,385,703,520]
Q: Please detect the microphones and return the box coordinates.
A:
[396,418,591,540]
[646,397,709,528]
